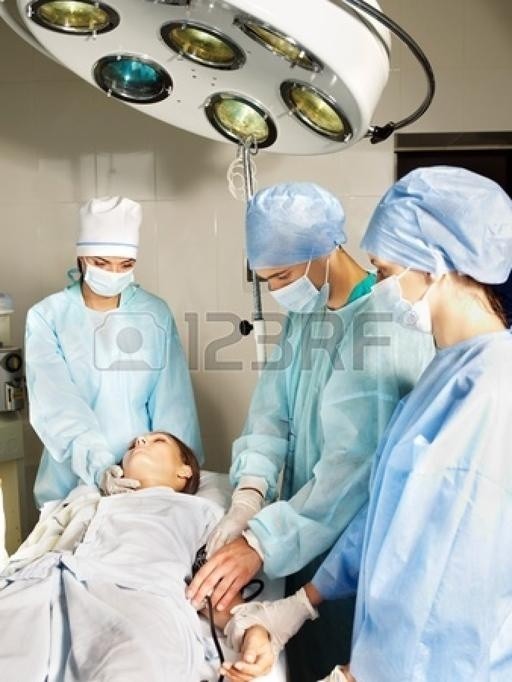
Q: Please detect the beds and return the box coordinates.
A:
[193,468,294,681]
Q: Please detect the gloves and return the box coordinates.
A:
[202,490,265,561]
[316,664,350,681]
[224,587,319,660]
[101,465,140,495]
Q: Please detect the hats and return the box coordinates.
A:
[76,195,142,261]
[245,181,348,269]
[359,164,512,284]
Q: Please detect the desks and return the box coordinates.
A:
[1,412,22,560]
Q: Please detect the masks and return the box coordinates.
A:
[370,265,444,333]
[83,255,135,297]
[267,252,330,315]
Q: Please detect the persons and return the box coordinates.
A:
[0,430,278,682]
[186,181,437,681]
[222,163,511,680]
[23,196,207,512]
